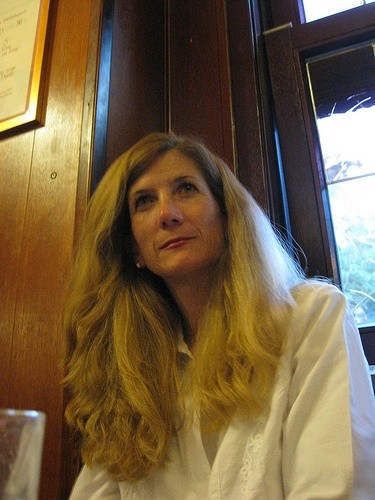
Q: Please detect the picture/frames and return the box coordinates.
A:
[1,0,56,140]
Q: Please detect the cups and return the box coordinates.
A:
[0,408,45,500]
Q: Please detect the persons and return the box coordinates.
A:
[59,133,375,500]
